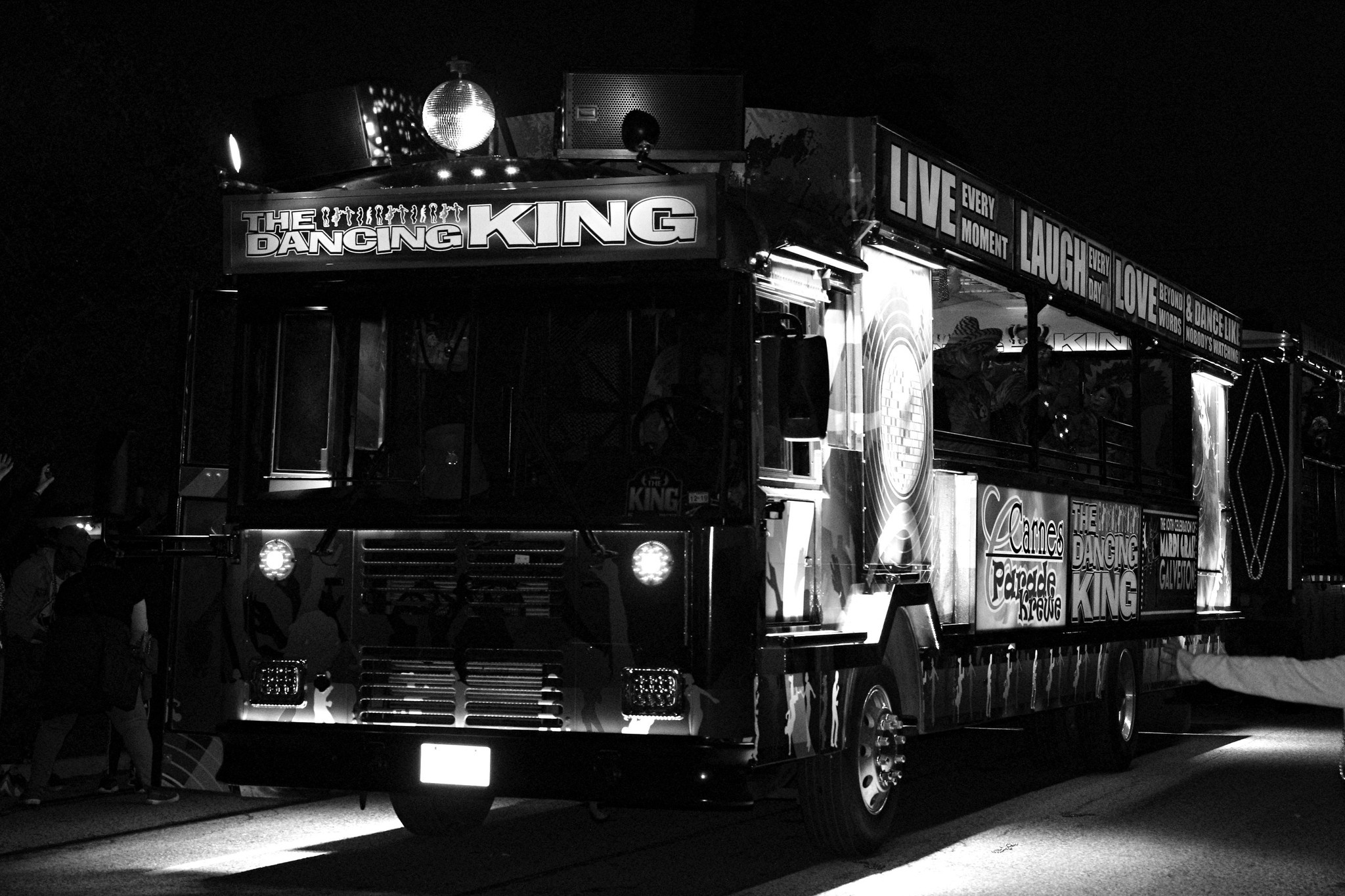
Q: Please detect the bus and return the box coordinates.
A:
[172,64,1345,862]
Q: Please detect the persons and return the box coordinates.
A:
[1161,637,1345,708]
[0,453,179,806]
[933,318,1126,481]
[637,291,741,444]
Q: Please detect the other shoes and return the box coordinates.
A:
[96,778,119,793]
[147,787,179,805]
[133,777,145,793]
[15,786,43,805]
[45,771,63,792]
